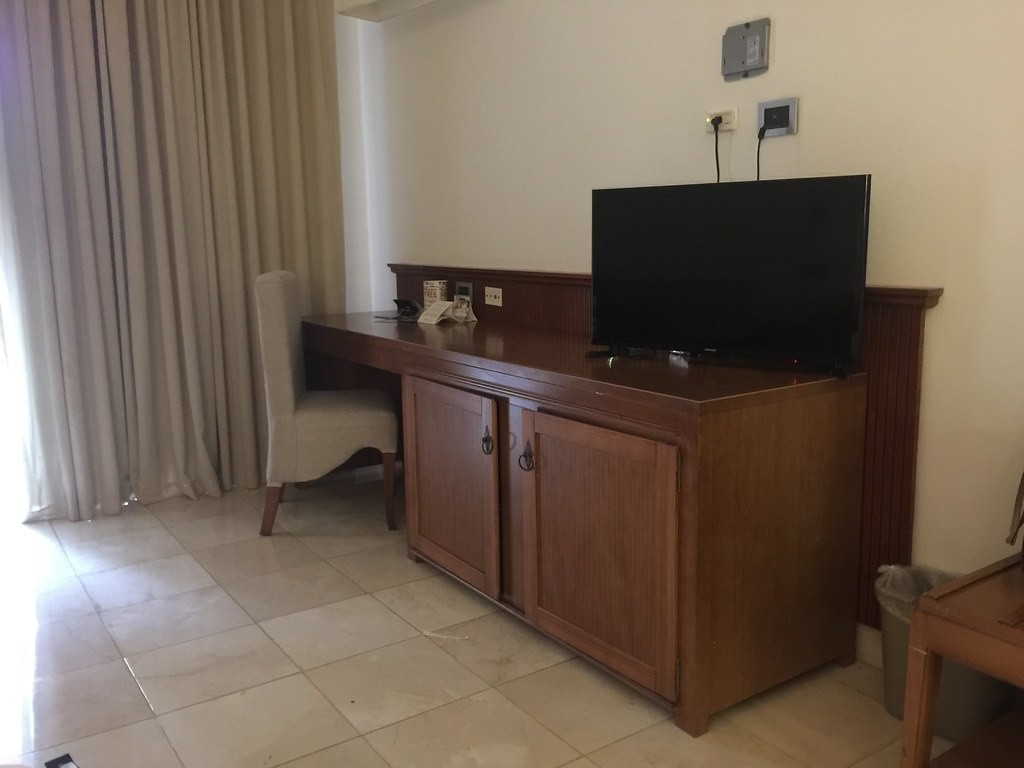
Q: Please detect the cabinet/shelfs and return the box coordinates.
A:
[401,334,868,739]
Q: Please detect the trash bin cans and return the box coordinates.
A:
[874,565,1013,743]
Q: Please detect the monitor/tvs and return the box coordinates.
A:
[590,174,872,376]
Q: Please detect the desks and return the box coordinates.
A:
[300,309,510,473]
[901,551,1023,768]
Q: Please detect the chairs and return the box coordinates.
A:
[255,270,399,536]
